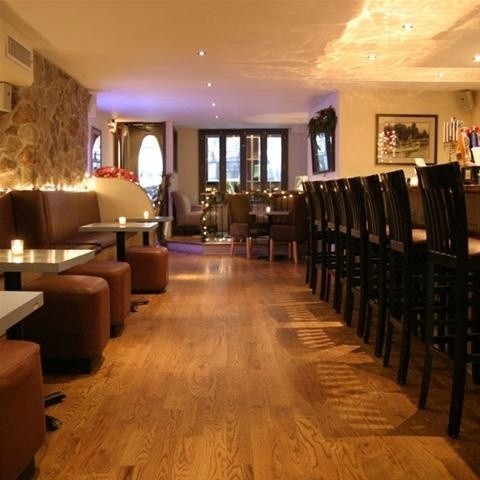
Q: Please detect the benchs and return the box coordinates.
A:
[9,190,138,262]
[171,189,219,235]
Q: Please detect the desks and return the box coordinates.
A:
[0,289,44,339]
[0,249,96,431]
[118,214,175,246]
[249,210,290,262]
[78,222,159,314]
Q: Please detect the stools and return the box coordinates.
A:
[0,339,48,480]
[21,274,110,376]
[60,261,131,338]
[125,245,170,293]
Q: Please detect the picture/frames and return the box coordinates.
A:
[375,115,438,165]
[310,126,334,174]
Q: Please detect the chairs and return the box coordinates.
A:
[345,176,389,336]
[227,193,270,259]
[335,178,361,321]
[380,170,457,383]
[305,181,322,288]
[416,162,480,438]
[270,183,305,264]
[312,181,335,302]
[361,174,429,356]
[324,180,346,309]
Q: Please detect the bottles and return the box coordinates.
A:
[471,127,477,146]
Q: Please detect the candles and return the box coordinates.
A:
[119,217,127,224]
[11,239,23,254]
[144,212,149,219]
